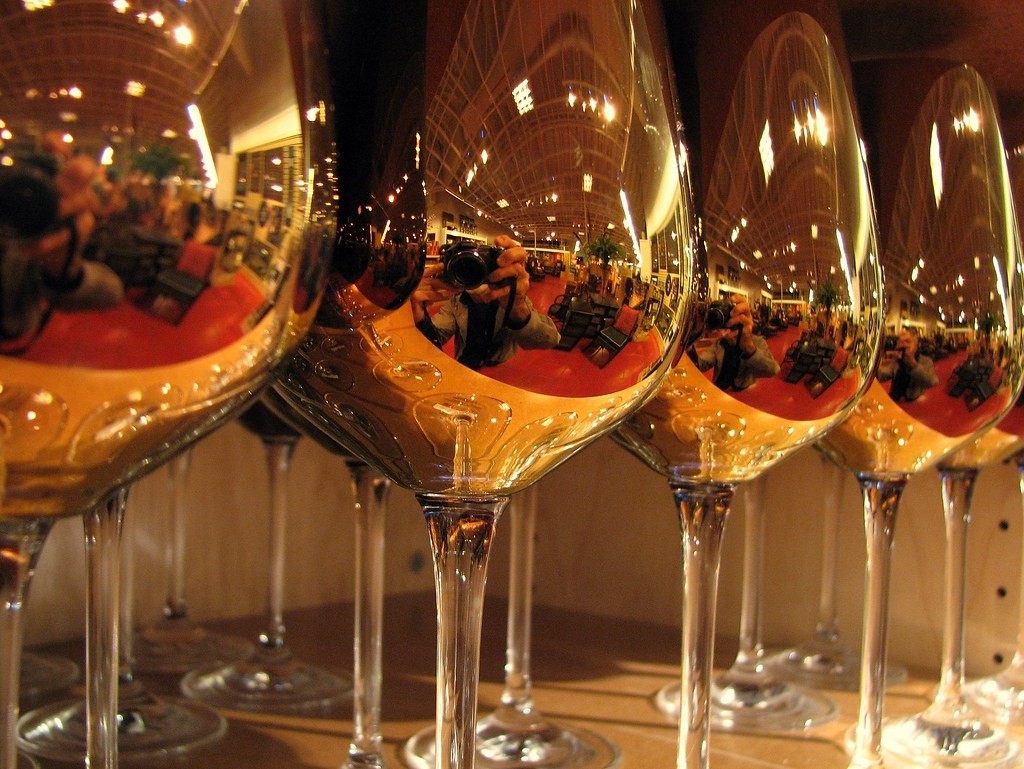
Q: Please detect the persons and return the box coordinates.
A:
[0,131,125,344]
[685,294,781,392]
[410,234,560,370]
[876,326,939,403]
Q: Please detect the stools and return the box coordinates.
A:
[139,267,207,328]
[803,364,840,400]
[581,326,628,368]
[964,380,993,413]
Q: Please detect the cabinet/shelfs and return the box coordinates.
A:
[581,288,620,339]
[553,296,594,353]
[806,338,837,375]
[783,342,817,385]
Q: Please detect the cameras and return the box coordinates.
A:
[0,151,64,240]
[439,240,516,290]
[708,301,742,331]
[884,336,905,353]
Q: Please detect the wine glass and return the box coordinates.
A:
[0,0,1023,769]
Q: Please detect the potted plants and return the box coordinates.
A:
[812,279,843,340]
[588,227,622,297]
